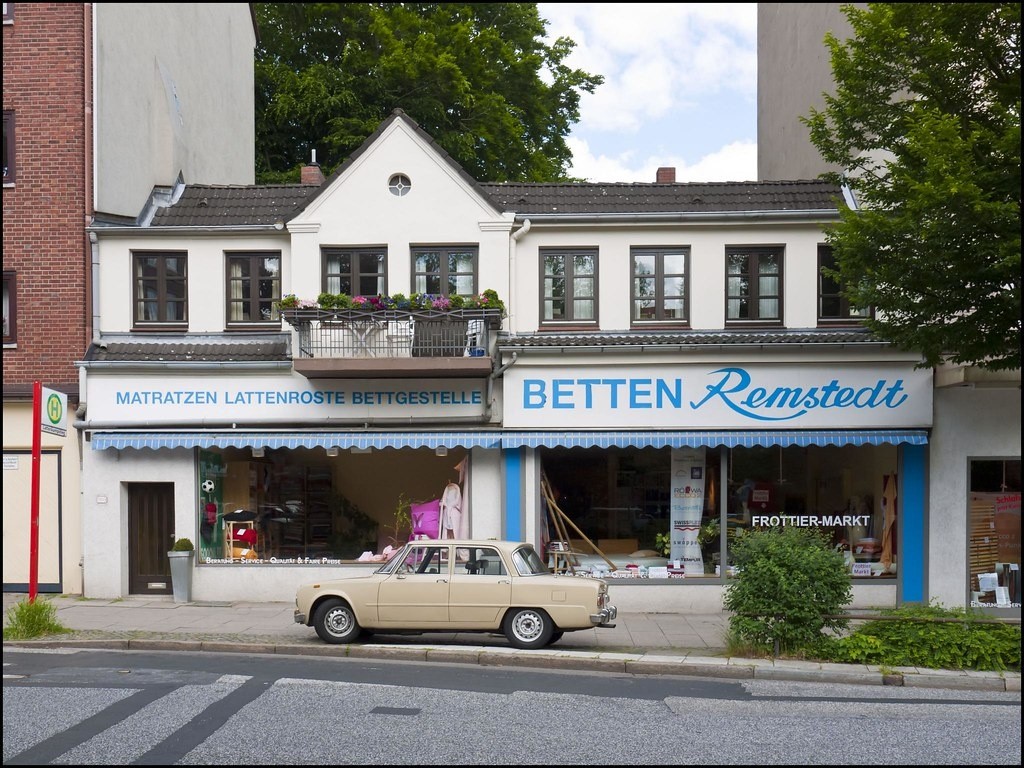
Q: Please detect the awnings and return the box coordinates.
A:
[86,429,931,450]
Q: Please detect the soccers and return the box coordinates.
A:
[202,480,215,493]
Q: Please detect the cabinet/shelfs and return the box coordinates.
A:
[220,461,280,559]
[223,520,255,560]
[279,460,335,550]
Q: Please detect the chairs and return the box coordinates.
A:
[465,560,482,575]
[430,568,439,574]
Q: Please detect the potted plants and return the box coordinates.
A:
[167,537,195,606]
[385,493,412,562]
[317,293,351,322]
[483,289,507,329]
[448,294,464,320]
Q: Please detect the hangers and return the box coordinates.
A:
[443,479,460,490]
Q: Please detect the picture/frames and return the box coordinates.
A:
[282,306,302,321]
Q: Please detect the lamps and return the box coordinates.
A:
[436,446,448,456]
[251,446,267,458]
[273,218,286,231]
[264,259,280,274]
[326,445,339,457]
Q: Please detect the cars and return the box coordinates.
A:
[293,540,618,649]
[259,504,306,539]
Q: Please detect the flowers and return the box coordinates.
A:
[695,522,717,547]
[654,530,671,557]
[471,293,488,308]
[300,297,319,309]
[274,289,299,314]
[352,293,452,312]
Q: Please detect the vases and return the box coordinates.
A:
[396,307,412,321]
[297,307,316,322]
[351,307,373,322]
[464,307,483,320]
[372,309,386,320]
[431,308,449,322]
[386,309,396,320]
[409,308,431,322]
